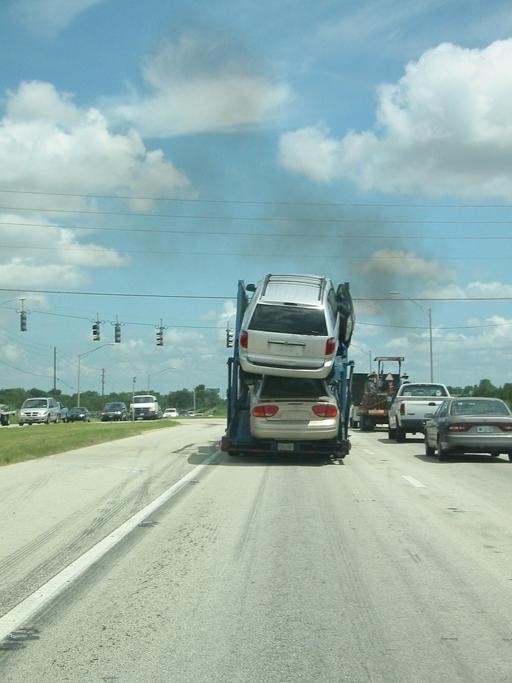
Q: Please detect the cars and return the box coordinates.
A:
[100,395,180,421]
[17,397,90,426]
[424,397,512,460]
[222,275,355,459]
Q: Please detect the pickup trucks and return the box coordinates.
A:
[389,383,453,443]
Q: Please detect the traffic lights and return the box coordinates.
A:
[92,325,100,340]
[156,331,164,346]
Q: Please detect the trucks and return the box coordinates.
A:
[350,372,383,428]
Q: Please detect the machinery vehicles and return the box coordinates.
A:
[359,357,411,431]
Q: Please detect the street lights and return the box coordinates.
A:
[147,366,176,395]
[387,290,434,383]
[77,342,118,407]
